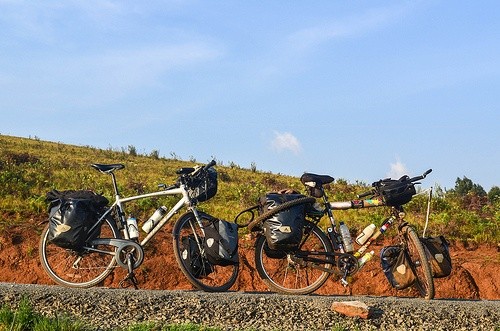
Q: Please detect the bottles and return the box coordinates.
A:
[355,223,377,245]
[127,215,139,239]
[141,206,168,233]
[358,250,375,267]
[338,220,354,252]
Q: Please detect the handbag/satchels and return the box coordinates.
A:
[381,245,418,290]
[182,235,214,280]
[264,241,298,259]
[420,235,452,277]
[177,167,217,203]
[375,175,416,206]
[202,218,239,267]
[46,188,109,254]
[264,192,308,251]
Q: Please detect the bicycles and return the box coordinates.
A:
[38,159,239,292]
[254,168,435,299]
